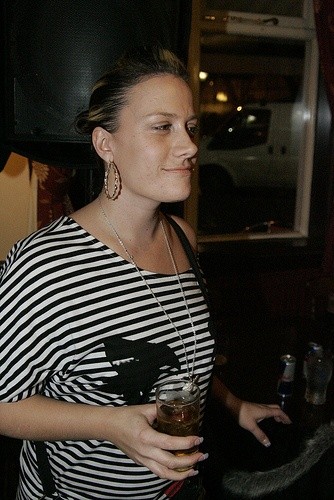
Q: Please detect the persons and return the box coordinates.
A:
[0,47,295,498]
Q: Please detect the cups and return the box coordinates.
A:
[154,378,202,473]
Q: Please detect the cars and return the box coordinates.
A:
[196,121,303,202]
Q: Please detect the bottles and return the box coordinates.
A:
[302,344,333,406]
[277,353,296,415]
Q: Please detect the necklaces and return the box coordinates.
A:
[98,194,201,396]
